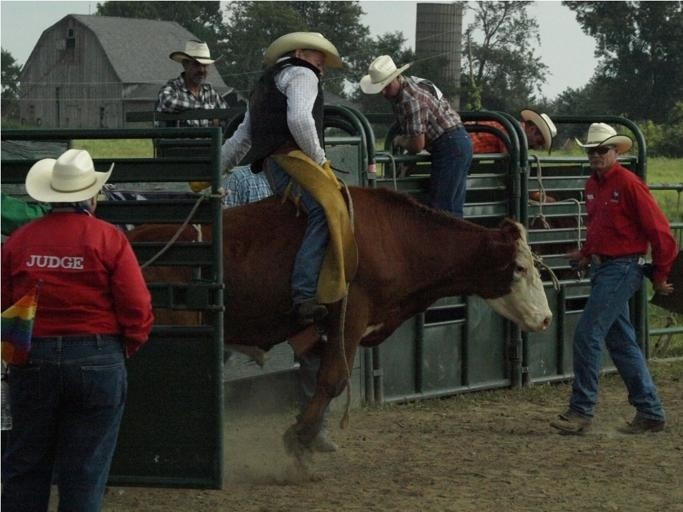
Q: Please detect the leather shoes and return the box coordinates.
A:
[550,409,592,433]
[617,413,666,435]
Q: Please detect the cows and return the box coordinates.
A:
[123,185,554,470]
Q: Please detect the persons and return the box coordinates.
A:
[1,148,155,511]
[360,54,472,220]
[550,122,678,434]
[152,39,231,130]
[466,108,558,153]
[191,30,343,324]
[221,162,274,209]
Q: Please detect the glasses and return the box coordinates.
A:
[585,147,615,155]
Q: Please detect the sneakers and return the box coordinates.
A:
[291,301,329,323]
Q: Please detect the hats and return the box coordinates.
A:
[520,108,557,153]
[359,54,410,95]
[574,121,634,155]
[24,148,115,203]
[169,39,224,66]
[264,31,343,70]
